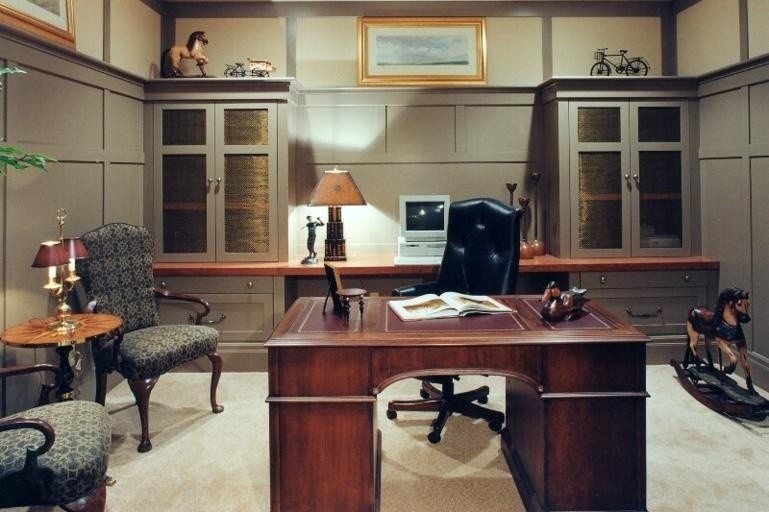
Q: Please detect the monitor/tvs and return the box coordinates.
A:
[395,193,450,264]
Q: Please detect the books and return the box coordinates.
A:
[388,291,518,321]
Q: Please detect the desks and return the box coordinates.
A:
[264,296,651,512]
[1,311,123,395]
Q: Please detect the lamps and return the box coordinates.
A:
[31,206,92,333]
[307,169,367,260]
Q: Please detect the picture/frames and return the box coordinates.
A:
[0,0,77,52]
[324,262,344,314]
[357,16,486,86]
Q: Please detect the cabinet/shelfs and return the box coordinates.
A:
[543,101,694,259]
[151,276,286,371]
[569,269,719,366]
[146,99,289,262]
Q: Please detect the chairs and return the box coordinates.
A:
[386,199,524,442]
[0,364,113,512]
[69,222,224,452]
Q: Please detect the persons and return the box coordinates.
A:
[300,215,325,257]
